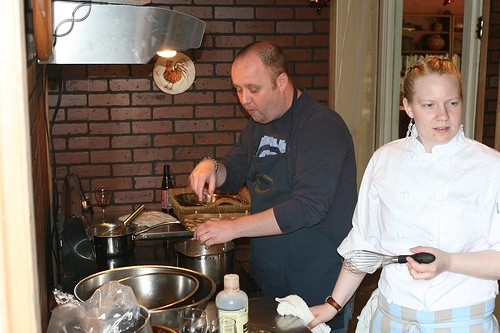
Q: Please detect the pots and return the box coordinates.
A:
[92,220,181,264]
[174,237,235,291]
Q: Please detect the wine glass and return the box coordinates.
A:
[95,186,112,223]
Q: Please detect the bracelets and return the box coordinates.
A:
[325,295,343,314]
[203,157,219,172]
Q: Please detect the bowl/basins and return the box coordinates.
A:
[73,265,217,333]
[173,192,207,207]
[95,272,200,311]
[115,305,153,333]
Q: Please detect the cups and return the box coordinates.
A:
[177,308,209,333]
[434,24,442,32]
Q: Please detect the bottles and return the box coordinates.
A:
[160,165,174,213]
[427,34,445,50]
[214,274,249,332]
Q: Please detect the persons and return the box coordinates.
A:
[306,55,500,333]
[189,41,359,333]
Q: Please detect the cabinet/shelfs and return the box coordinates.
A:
[401,15,455,105]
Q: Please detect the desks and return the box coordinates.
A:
[60,221,309,333]
